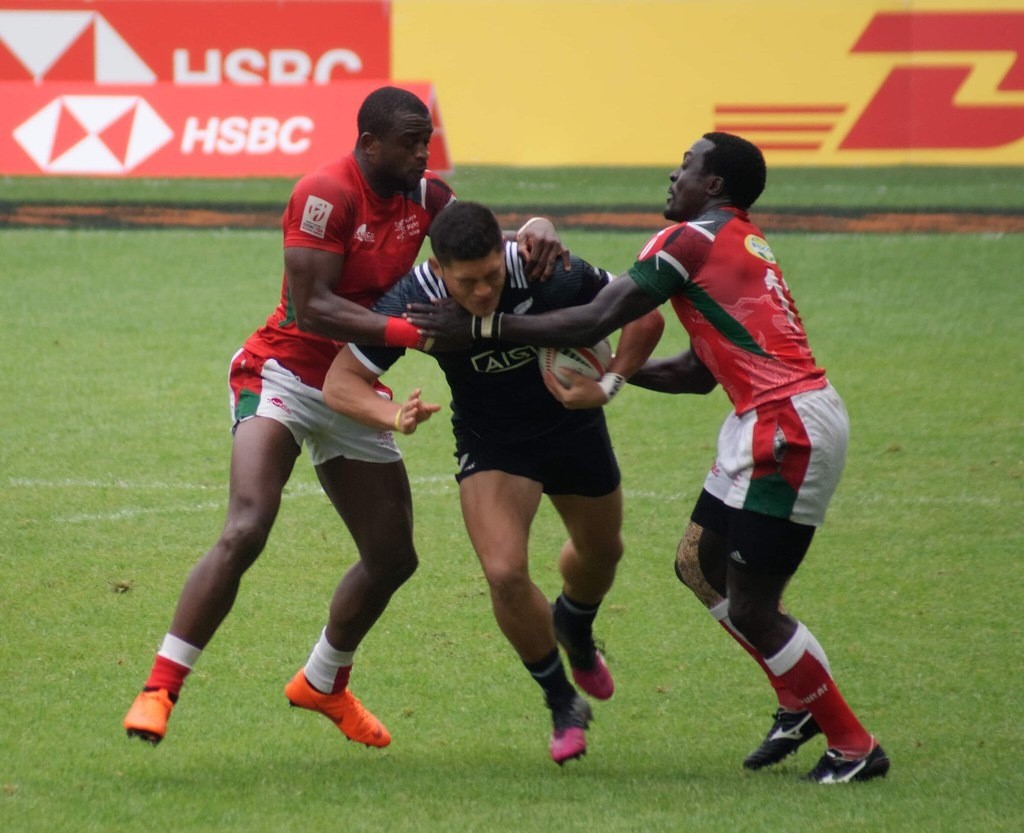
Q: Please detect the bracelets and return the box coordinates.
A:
[395,409,403,431]
[472,311,504,338]
[516,217,547,242]
[597,372,626,402]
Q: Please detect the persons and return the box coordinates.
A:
[125,87,572,746]
[403,132,889,786]
[321,200,666,765]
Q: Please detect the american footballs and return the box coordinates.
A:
[538,337,613,393]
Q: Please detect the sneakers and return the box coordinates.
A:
[743,705,822,771]
[549,694,593,766]
[285,669,391,748]
[550,604,614,700]
[800,744,891,785]
[123,685,178,747]
